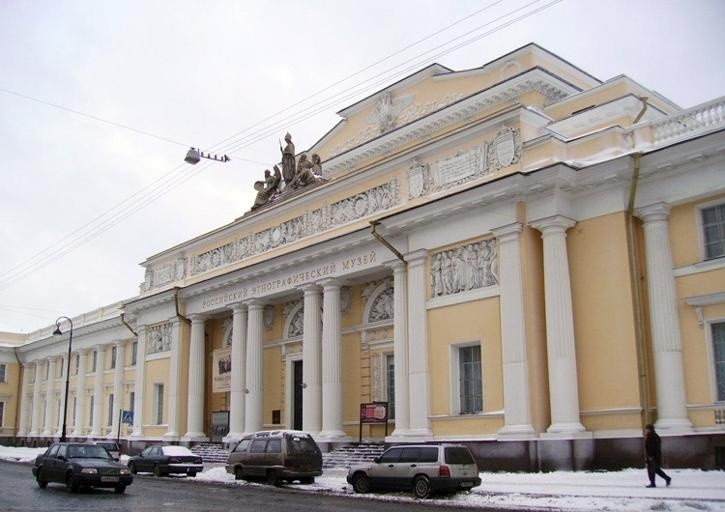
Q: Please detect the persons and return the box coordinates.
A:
[644,425,671,488]
[250,130,329,213]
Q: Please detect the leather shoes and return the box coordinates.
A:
[646,483,657,488]
[665,477,671,487]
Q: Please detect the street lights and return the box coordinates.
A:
[51,315,74,442]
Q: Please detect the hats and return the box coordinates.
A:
[644,424,655,430]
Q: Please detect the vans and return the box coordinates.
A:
[87,439,121,463]
[224,429,323,485]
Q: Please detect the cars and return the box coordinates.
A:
[32,442,133,493]
[347,444,481,500]
[127,445,204,477]
